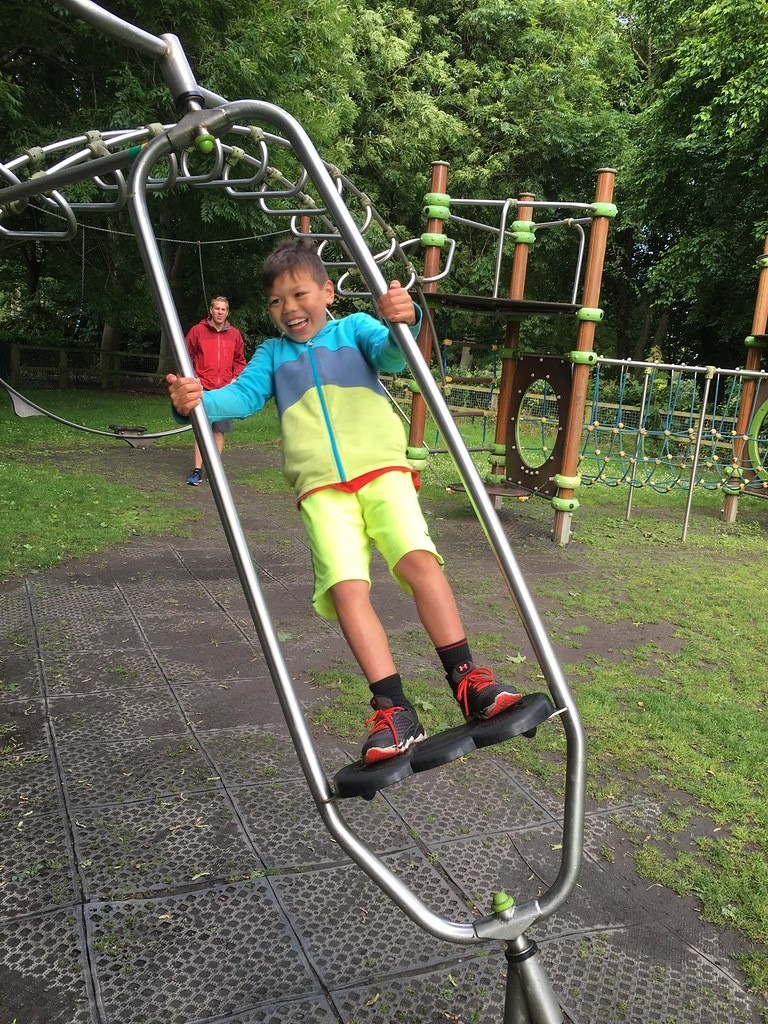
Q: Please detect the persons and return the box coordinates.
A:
[185,296,246,486]
[167,239,523,763]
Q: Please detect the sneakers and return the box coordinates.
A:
[362,694,428,763]
[186,469,203,486]
[446,660,523,721]
